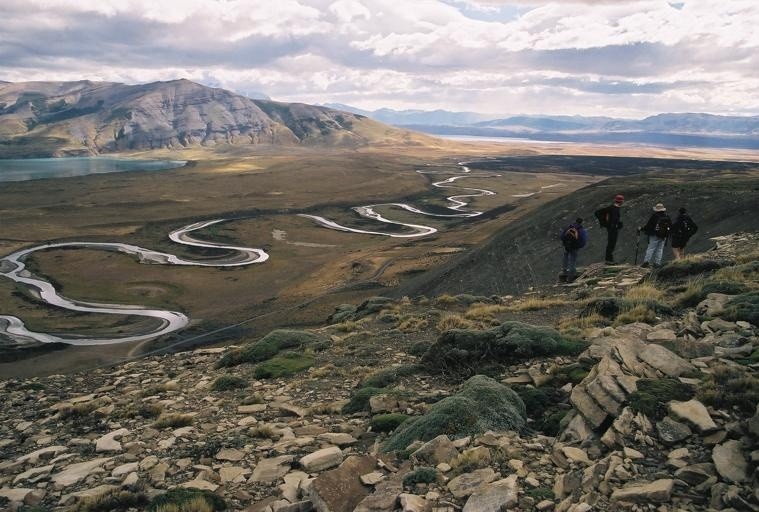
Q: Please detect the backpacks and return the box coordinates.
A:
[656,214,672,237]
[564,225,582,243]
[595,206,613,227]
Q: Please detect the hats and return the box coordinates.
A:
[653,204,666,211]
[615,194,624,203]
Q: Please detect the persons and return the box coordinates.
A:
[603,194,624,266]
[669,207,698,261]
[560,218,587,272]
[640,203,672,268]
